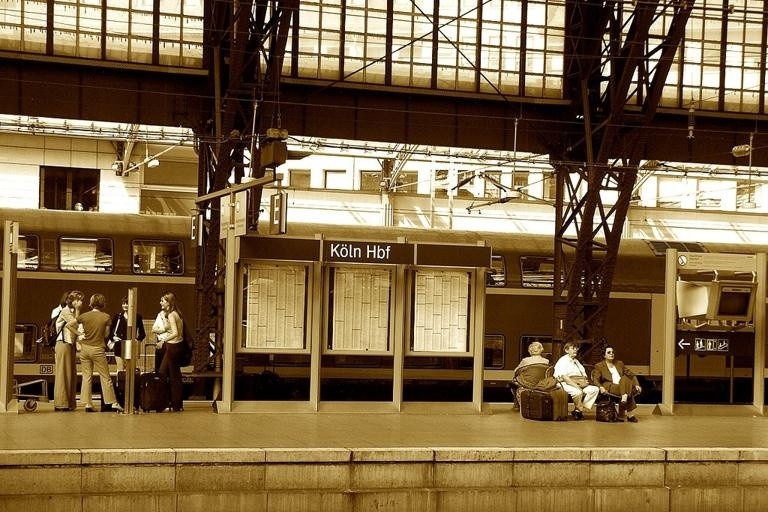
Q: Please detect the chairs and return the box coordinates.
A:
[509,363,549,408]
[546,364,595,403]
[590,369,639,403]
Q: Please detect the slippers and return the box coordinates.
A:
[86,404,97,412]
[112,403,124,412]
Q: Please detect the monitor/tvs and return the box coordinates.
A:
[677,280,713,320]
[705,280,757,321]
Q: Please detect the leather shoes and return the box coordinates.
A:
[627,416,638,422]
[571,411,584,420]
[620,401,627,410]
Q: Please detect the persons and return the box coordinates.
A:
[510,341,549,410]
[151,302,174,371]
[49,289,146,415]
[551,339,600,422]
[591,344,643,423]
[154,291,185,412]
[72,201,86,211]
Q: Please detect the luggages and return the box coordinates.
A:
[117,344,166,412]
[521,377,569,421]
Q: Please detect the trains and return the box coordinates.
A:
[0,204,768,408]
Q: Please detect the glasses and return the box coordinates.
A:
[607,351,614,354]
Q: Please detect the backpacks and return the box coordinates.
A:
[43,310,67,347]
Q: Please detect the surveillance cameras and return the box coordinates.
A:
[147,159,160,168]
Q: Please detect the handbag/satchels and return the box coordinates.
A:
[180,341,193,367]
[570,375,589,389]
[107,333,122,350]
[597,400,618,422]
